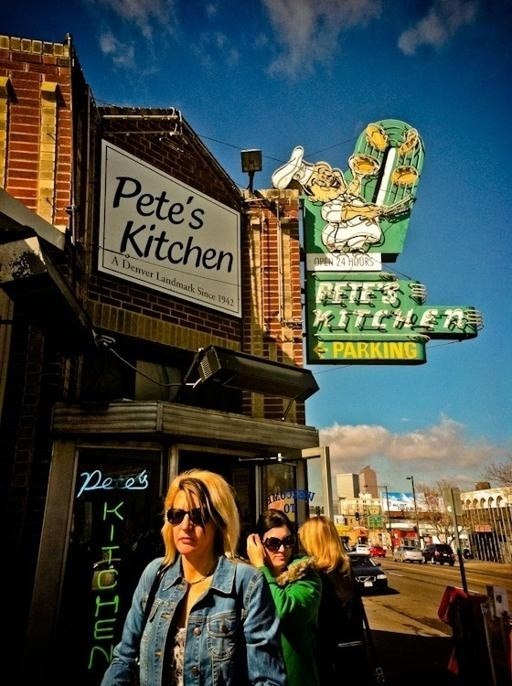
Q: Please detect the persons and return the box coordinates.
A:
[245,507,323,683]
[99,468,289,686]
[297,514,364,686]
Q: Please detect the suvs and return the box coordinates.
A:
[422,542,460,567]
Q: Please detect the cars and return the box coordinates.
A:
[345,553,389,595]
[394,547,426,563]
[353,543,388,559]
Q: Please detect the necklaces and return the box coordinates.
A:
[184,564,218,585]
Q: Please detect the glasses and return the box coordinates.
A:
[166,507,215,526]
[263,534,297,552]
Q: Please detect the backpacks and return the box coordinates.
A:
[318,571,381,685]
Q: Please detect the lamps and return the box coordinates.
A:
[239,147,264,196]
[172,343,321,423]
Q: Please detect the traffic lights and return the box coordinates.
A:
[355,511,359,520]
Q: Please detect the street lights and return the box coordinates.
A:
[405,474,422,551]
[363,483,394,545]
